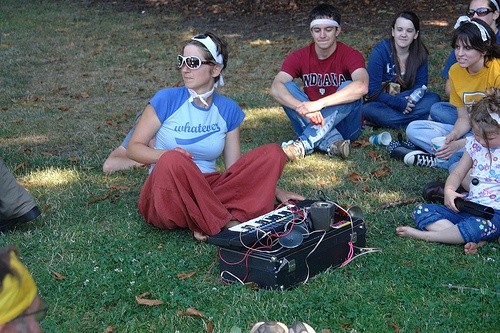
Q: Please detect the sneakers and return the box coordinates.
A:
[404,150,436,167]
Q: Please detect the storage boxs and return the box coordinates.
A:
[222,218,365,292]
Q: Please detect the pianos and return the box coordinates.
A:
[207,198,321,248]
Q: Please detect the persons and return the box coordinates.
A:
[362,12,441,130]
[0,244,43,333]
[103,111,155,174]
[126,31,305,240]
[395,0,500,255]
[0,157,42,231]
[269,3,369,162]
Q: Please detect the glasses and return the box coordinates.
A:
[177,54,213,70]
[465,7,494,18]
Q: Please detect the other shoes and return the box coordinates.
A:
[282,140,305,161]
[0,206,41,232]
[327,140,350,159]
[422,181,445,203]
